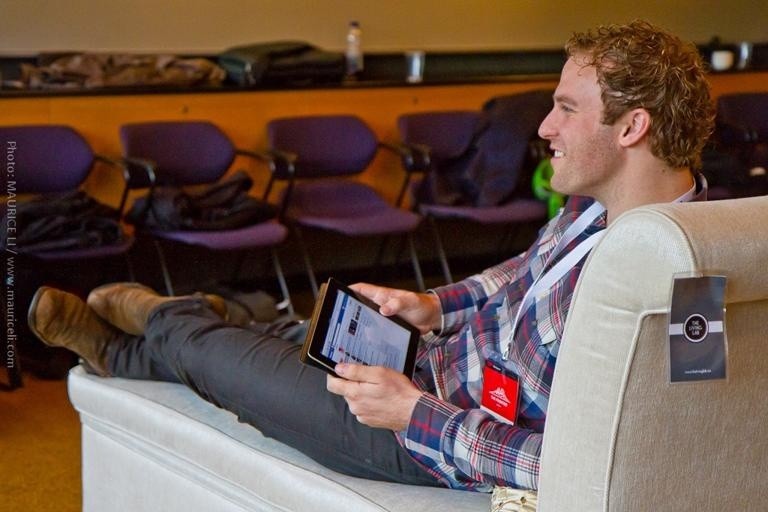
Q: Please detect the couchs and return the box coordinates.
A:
[66,196,768,512]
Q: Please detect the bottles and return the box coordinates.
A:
[345,21,364,75]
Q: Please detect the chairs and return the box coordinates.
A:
[1,88,555,387]
[705,89,767,195]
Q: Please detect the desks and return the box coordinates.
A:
[0,71,768,293]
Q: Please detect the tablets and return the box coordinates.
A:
[307,277,421,385]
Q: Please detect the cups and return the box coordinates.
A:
[399,49,426,84]
[710,50,734,71]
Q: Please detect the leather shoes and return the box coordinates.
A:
[27,287,125,375]
[86,282,228,336]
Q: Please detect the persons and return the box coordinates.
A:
[27,15,720,502]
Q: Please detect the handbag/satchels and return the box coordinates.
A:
[425,115,538,207]
[17,53,226,94]
[220,41,346,87]
